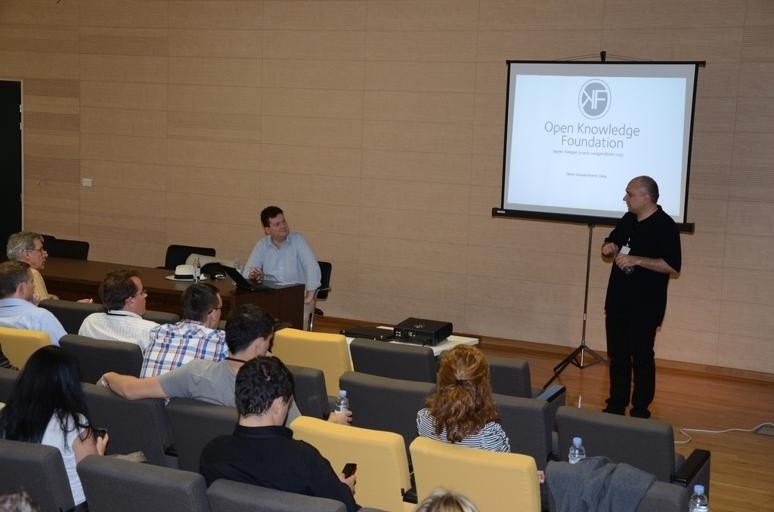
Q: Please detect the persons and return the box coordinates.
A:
[78,268,161,360]
[140,284,229,405]
[93,303,352,434]
[602,176,682,419]
[414,490,480,512]
[415,344,544,488]
[241,206,322,331]
[0,260,68,347]
[0,345,109,512]
[6,231,93,308]
[197,354,362,511]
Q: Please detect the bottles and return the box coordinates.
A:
[568,438,587,465]
[334,389,350,414]
[611,248,634,274]
[688,485,710,512]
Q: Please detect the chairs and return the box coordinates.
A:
[0,299,714,510]
[304,260,332,333]
[42,236,90,262]
[157,244,216,272]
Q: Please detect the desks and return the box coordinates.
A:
[0,259,306,330]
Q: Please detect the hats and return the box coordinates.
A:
[164,264,201,283]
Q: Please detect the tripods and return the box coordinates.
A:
[540,221,610,392]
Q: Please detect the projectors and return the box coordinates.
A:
[393,317,453,348]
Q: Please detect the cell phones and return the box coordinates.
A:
[342,463,358,478]
[94,427,107,439]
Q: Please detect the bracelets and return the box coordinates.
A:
[100,376,107,387]
